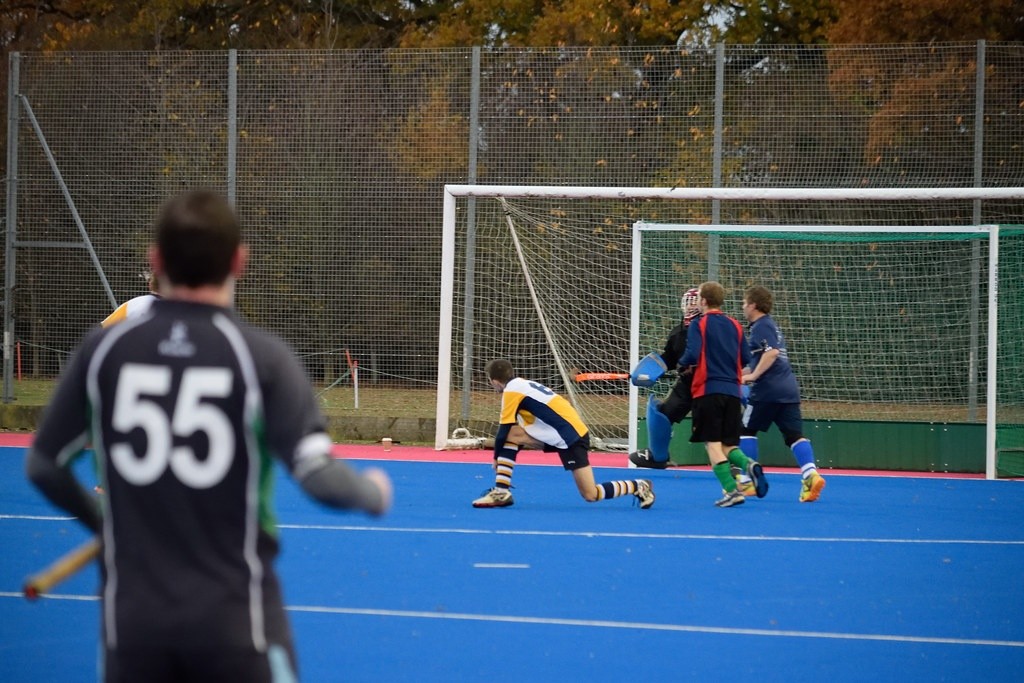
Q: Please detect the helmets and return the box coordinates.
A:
[679,288,702,326]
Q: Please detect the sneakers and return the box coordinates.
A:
[472,487,513,507]
[734,475,756,496]
[748,461,768,498]
[633,479,655,509]
[799,471,826,503]
[629,448,659,469]
[715,489,746,508]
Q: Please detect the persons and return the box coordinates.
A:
[629,287,752,479]
[741,287,826,502]
[26,191,393,683]
[676,281,769,507]
[471,359,656,509]
[94,272,163,494]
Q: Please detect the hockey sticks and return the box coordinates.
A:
[22,533,100,598]
[568,366,676,381]
